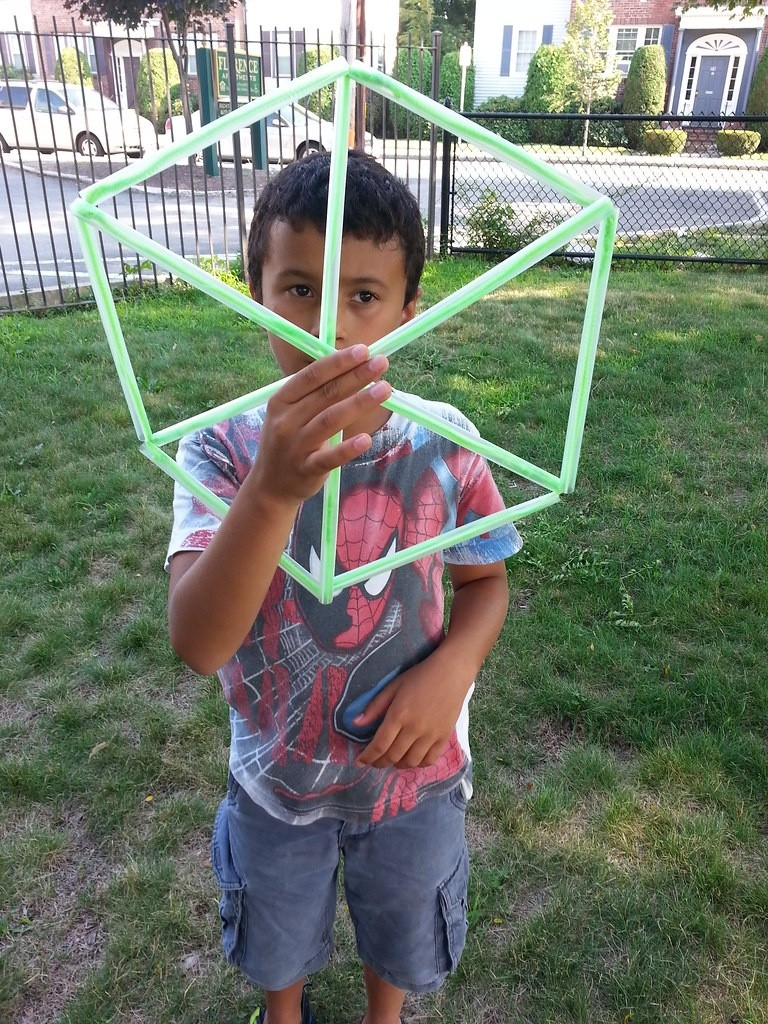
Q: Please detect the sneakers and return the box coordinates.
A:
[246,990,313,1024]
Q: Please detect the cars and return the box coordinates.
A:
[0,80,156,159]
[164,99,379,165]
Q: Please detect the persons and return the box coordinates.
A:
[164,149,524,1024]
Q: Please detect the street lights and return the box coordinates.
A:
[456,41,473,147]
